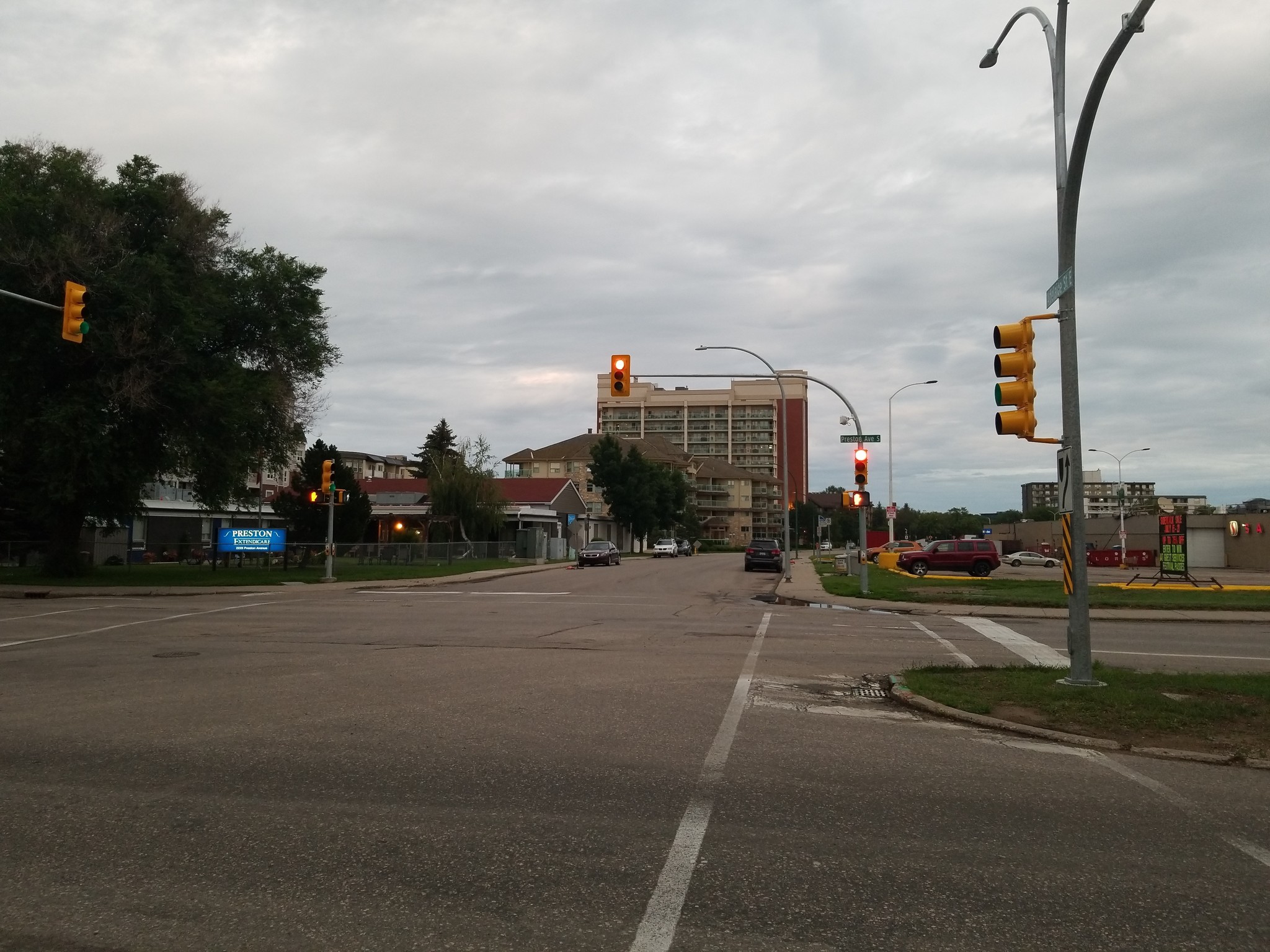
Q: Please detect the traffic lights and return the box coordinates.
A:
[611,354,631,397]
[321,461,336,495]
[852,490,870,507]
[854,448,869,485]
[992,322,1039,442]
[62,280,92,344]
[308,490,324,504]
[841,493,850,509]
[339,490,352,506]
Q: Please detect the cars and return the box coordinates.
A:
[1002,551,1061,568]
[866,540,926,564]
[1243,497,1270,506]
[578,540,621,567]
[845,543,856,550]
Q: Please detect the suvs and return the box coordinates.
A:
[675,538,693,557]
[653,538,678,558]
[744,536,784,573]
[820,542,832,551]
[896,537,1001,577]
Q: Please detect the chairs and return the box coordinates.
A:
[379,548,393,565]
[360,548,375,565]
[395,547,409,566]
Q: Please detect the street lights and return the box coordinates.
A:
[888,380,939,545]
[1047,510,1059,521]
[764,462,799,560]
[1088,448,1152,564]
[694,344,794,583]
[1092,508,1111,515]
